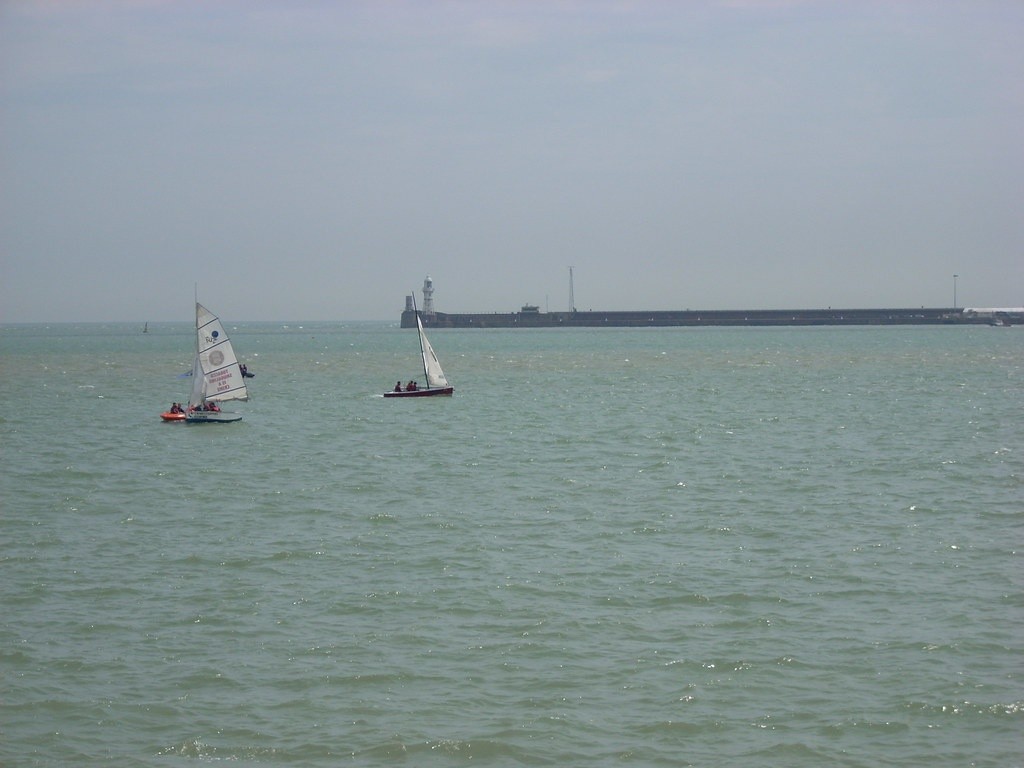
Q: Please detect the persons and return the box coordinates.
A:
[394,380,418,391]
[170,364,247,414]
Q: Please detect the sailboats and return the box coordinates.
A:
[185,281,249,423]
[381,312,454,398]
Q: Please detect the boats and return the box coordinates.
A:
[160,411,186,421]
